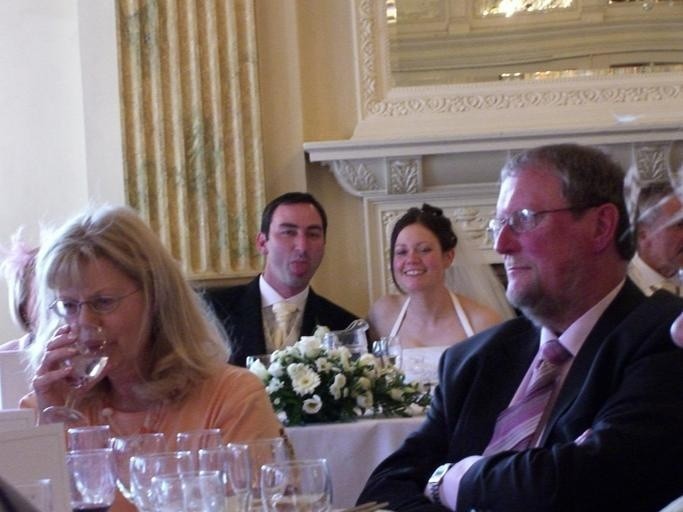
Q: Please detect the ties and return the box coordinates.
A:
[480,339,573,458]
[268,300,300,354]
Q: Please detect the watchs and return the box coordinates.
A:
[427,462,455,506]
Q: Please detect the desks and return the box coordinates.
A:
[273,403,429,510]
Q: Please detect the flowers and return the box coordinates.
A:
[246,321,434,426]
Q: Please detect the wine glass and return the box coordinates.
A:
[42,321,113,426]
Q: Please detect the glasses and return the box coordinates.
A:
[47,286,144,318]
[485,207,575,241]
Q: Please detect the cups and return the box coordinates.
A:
[4,478,54,512]
[323,327,401,374]
[245,353,272,367]
[66,426,331,511]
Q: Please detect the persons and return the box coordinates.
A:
[0,223,41,352]
[195,192,373,369]
[354,143,683,512]
[18,204,298,512]
[365,203,505,395]
[627,179,683,299]
